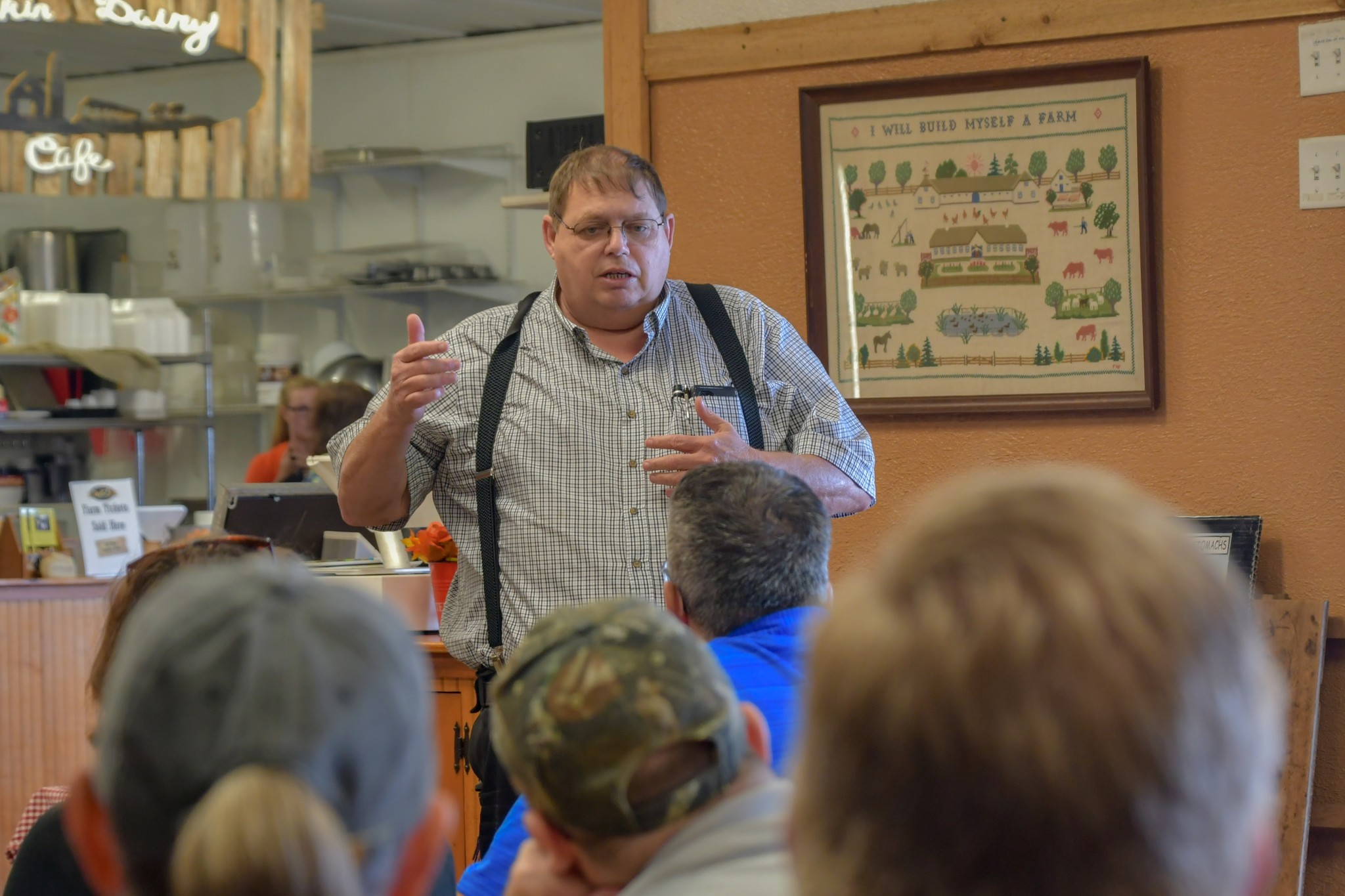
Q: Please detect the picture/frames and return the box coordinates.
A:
[795,56,1160,419]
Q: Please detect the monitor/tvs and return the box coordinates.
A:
[213,482,385,557]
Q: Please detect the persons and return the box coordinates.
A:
[87,552,458,896]
[484,597,799,895]
[3,525,320,896]
[239,374,377,484]
[319,144,883,874]
[464,455,846,895]
[799,462,1293,896]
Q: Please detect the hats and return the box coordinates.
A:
[489,596,745,839]
[97,560,439,894]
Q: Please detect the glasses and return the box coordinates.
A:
[286,404,313,416]
[552,209,665,242]
[125,535,275,596]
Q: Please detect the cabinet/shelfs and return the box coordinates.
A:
[408,631,484,891]
[175,154,554,422]
[2,300,213,894]
